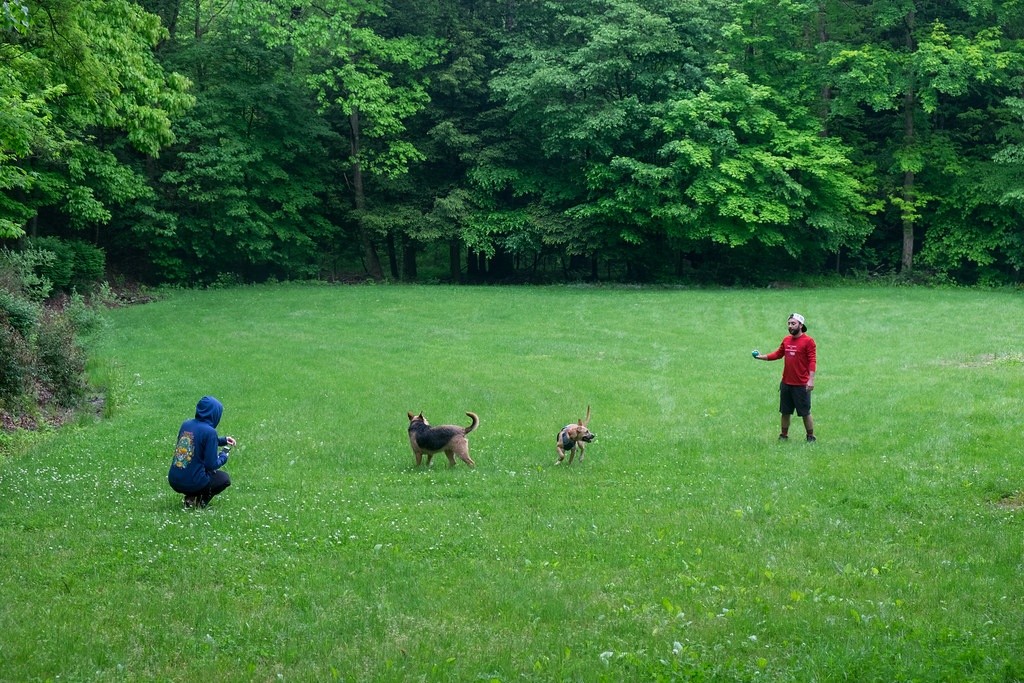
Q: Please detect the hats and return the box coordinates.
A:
[788,313,807,332]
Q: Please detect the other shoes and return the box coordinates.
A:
[806,435,816,441]
[182,496,198,506]
[778,434,789,440]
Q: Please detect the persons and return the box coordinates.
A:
[168,396,236,510]
[754,313,817,442]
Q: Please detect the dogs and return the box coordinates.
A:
[408,410,479,465]
[555,405,594,465]
